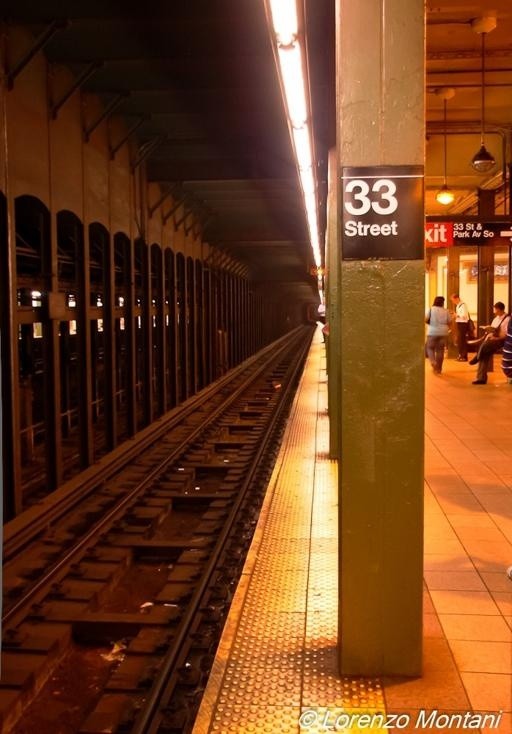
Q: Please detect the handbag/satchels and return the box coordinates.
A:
[467,320,475,332]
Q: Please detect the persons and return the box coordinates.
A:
[322,322,329,336]
[317,296,325,343]
[500,312,512,380]
[469,302,511,386]
[450,294,471,362]
[425,297,452,375]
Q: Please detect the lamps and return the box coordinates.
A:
[434,14,498,205]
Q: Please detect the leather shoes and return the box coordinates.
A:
[472,380,487,384]
[469,356,479,365]
